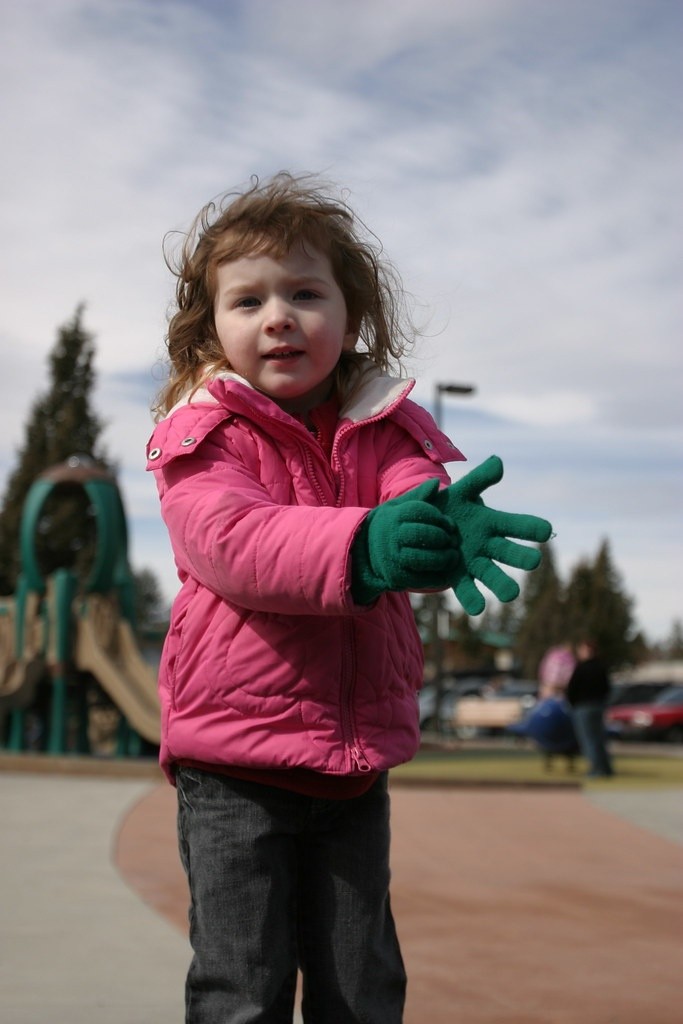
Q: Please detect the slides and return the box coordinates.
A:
[70,594,162,745]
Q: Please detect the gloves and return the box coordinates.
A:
[349,478,462,606]
[426,454,553,615]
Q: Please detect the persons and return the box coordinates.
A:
[537,632,613,777]
[146,176,553,1024]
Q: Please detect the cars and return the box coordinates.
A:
[605,684,683,743]
[419,672,540,738]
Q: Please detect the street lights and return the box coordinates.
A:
[431,382,475,737]
